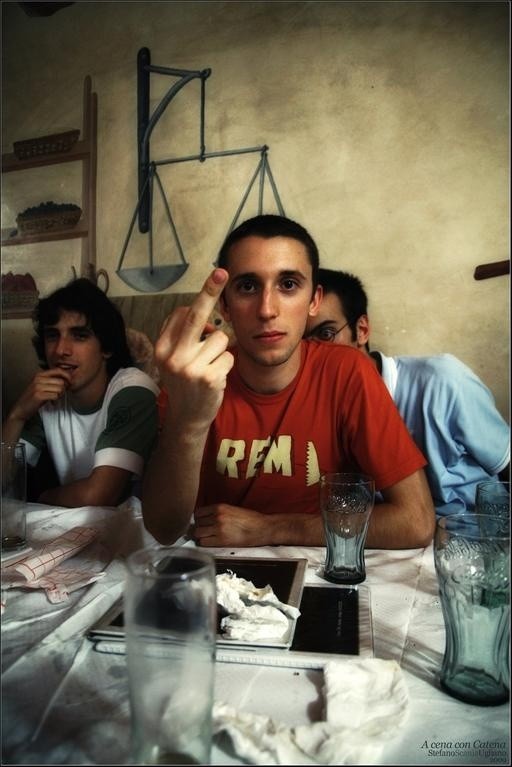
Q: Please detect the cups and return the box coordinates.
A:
[433,481,511,708]
[322,474,375,585]
[0,443,28,553]
[122,546,213,767]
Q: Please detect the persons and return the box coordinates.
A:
[0,277,162,508]
[132,212,436,550]
[298,266,511,544]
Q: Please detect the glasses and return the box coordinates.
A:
[317,322,348,344]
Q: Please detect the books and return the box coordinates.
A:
[85,550,310,654]
[93,581,376,671]
